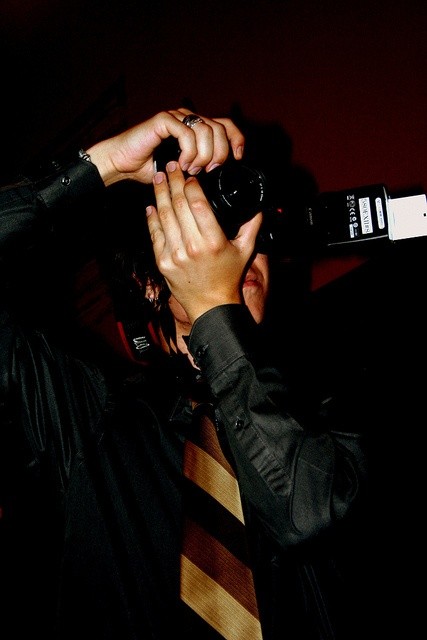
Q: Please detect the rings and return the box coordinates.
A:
[182,114,204,127]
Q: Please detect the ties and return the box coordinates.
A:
[182,397,262,640]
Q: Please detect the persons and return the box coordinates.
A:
[1,108,378,633]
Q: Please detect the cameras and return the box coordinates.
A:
[153,136,390,258]
